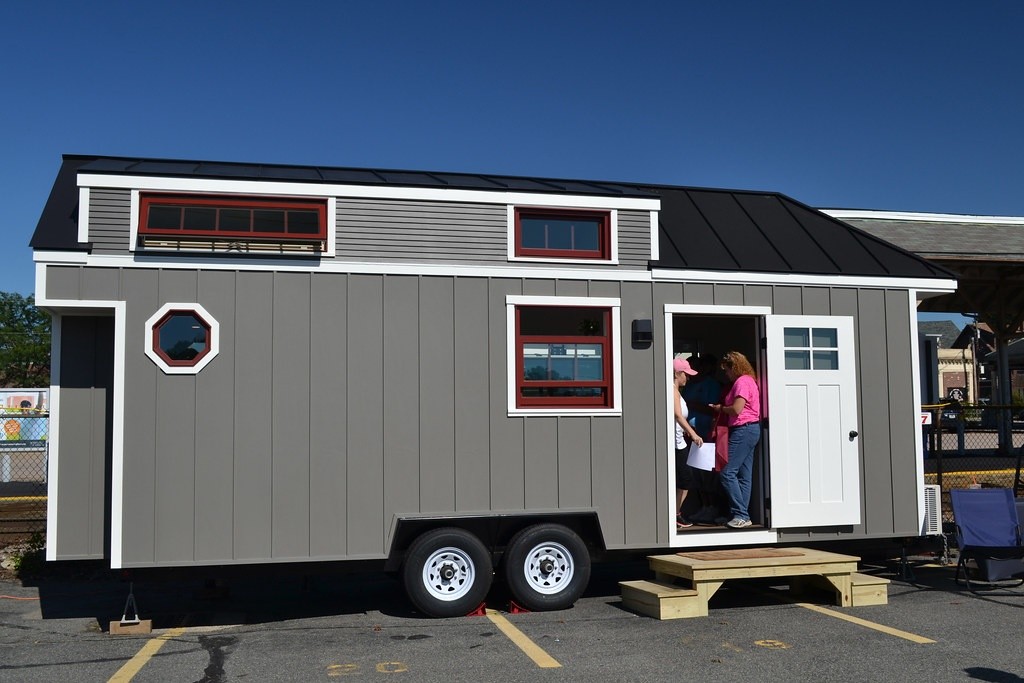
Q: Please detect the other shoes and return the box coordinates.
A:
[676,513,693,529]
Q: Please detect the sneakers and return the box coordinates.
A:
[727,517,752,528]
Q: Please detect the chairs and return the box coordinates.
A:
[949,487,1024,596]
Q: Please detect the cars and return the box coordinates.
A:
[977,398,1024,430]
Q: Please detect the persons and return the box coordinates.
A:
[674,358,704,528]
[709,351,761,527]
[17,391,43,440]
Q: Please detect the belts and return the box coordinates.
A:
[733,421,759,428]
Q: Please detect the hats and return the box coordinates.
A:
[673,358,698,375]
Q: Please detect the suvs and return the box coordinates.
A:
[938,397,965,434]
